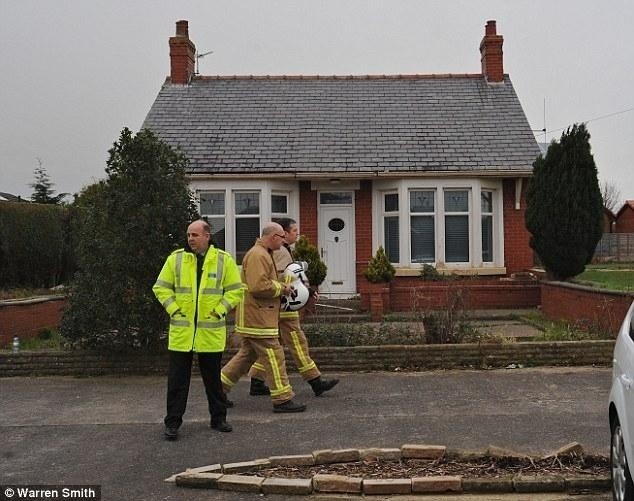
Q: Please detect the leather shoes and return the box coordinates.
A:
[211,417,232,432]
[165,426,178,438]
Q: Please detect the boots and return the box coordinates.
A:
[309,377,339,396]
[250,378,270,395]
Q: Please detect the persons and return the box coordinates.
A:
[219,223,307,413]
[153,218,244,440]
[248,218,338,396]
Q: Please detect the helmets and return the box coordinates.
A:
[284,263,304,282]
[280,278,310,311]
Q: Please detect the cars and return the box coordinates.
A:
[607,299,634,501]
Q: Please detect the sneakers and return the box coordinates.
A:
[223,394,233,407]
[273,400,307,412]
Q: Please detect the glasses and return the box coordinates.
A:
[275,234,285,239]
